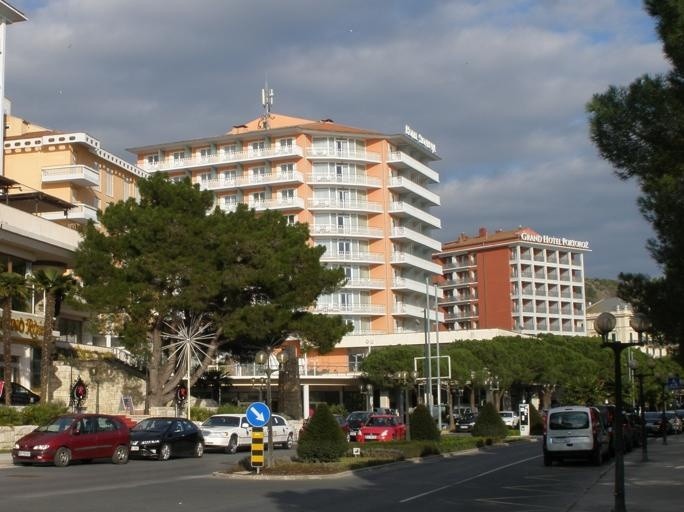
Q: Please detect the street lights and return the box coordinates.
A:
[392,371,419,441]
[629,358,656,463]
[654,372,673,445]
[254,350,289,469]
[593,312,649,512]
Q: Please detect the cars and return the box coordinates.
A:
[498,411,519,429]
[0,380,41,404]
[357,415,406,443]
[299,415,351,443]
[433,404,479,432]
[129,417,206,462]
[346,411,377,440]
[198,413,294,455]
[546,405,684,466]
[11,413,139,467]
